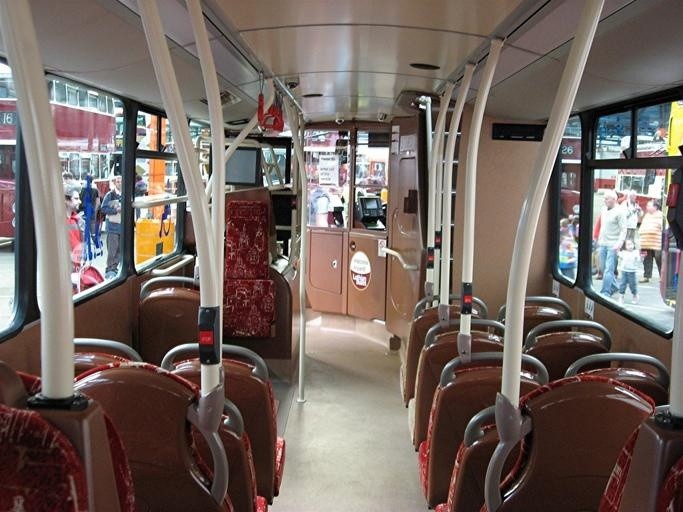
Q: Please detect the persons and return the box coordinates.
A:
[559,189,663,304]
[62,171,147,296]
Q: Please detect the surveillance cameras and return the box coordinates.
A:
[286,79,299,89]
[377,112,387,122]
[336,119,344,124]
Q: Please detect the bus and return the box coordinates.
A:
[302,145,386,230]
[0,96,210,248]
[559,97,683,311]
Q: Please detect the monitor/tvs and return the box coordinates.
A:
[209,144,262,187]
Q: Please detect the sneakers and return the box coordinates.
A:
[617,294,625,305]
[637,277,650,283]
[631,293,640,305]
[595,270,620,298]
